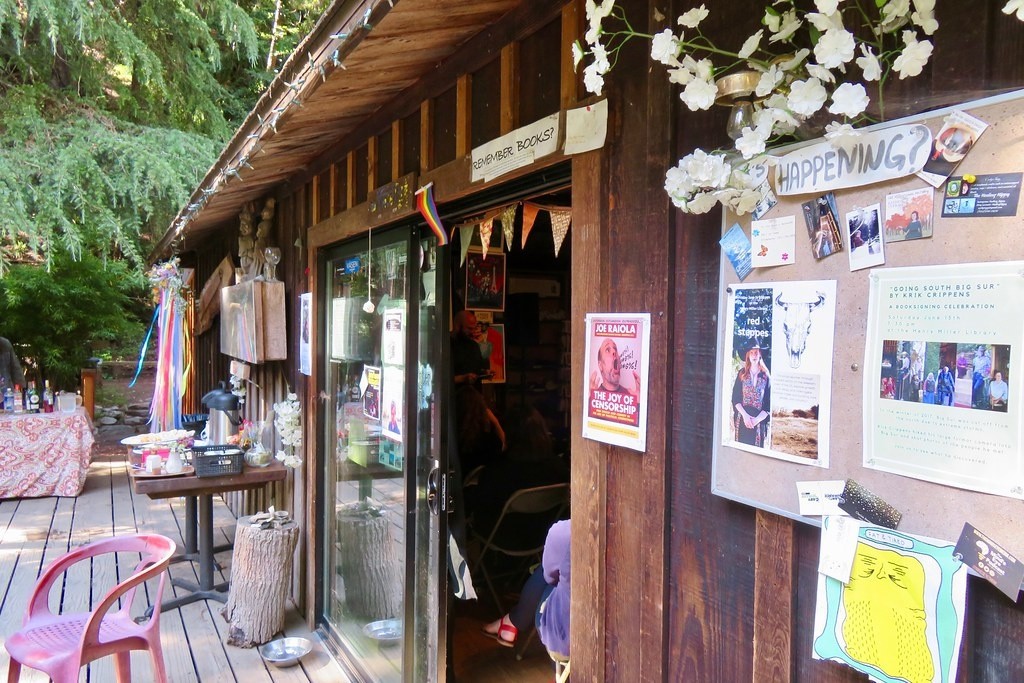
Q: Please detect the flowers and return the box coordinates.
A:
[144,253,194,316]
[0,371,8,392]
[571,0,1024,214]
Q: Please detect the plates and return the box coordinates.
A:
[132,442,191,454]
[120,432,193,448]
[129,466,195,477]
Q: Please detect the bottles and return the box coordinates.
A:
[3,377,39,413]
[166,444,182,474]
[42,380,53,413]
[244,421,273,468]
[145,450,161,475]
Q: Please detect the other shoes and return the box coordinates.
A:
[972,402,977,407]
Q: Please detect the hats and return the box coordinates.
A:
[743,337,761,348]
[902,351,908,355]
[979,346,986,351]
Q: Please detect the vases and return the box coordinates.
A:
[0,387,5,403]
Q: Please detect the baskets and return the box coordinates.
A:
[191,444,245,477]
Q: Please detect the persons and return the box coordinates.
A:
[450,311,496,394]
[732,336,770,449]
[450,387,570,599]
[882,345,1008,411]
[0,336,27,410]
[588,338,640,427]
[388,401,400,435]
[479,519,571,663]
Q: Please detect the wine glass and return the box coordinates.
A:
[265,247,281,281]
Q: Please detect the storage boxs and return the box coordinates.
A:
[191,445,245,478]
[347,441,379,468]
[58,397,77,411]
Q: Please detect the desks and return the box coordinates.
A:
[126,438,288,618]
[0,404,98,499]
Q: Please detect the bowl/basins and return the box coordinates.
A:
[260,637,314,668]
[362,620,402,646]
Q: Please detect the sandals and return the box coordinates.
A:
[478,614,517,648]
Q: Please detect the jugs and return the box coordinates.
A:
[200,388,243,445]
[60,392,82,414]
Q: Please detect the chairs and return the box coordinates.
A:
[469,484,571,620]
[4,532,177,683]
[516,624,570,683]
